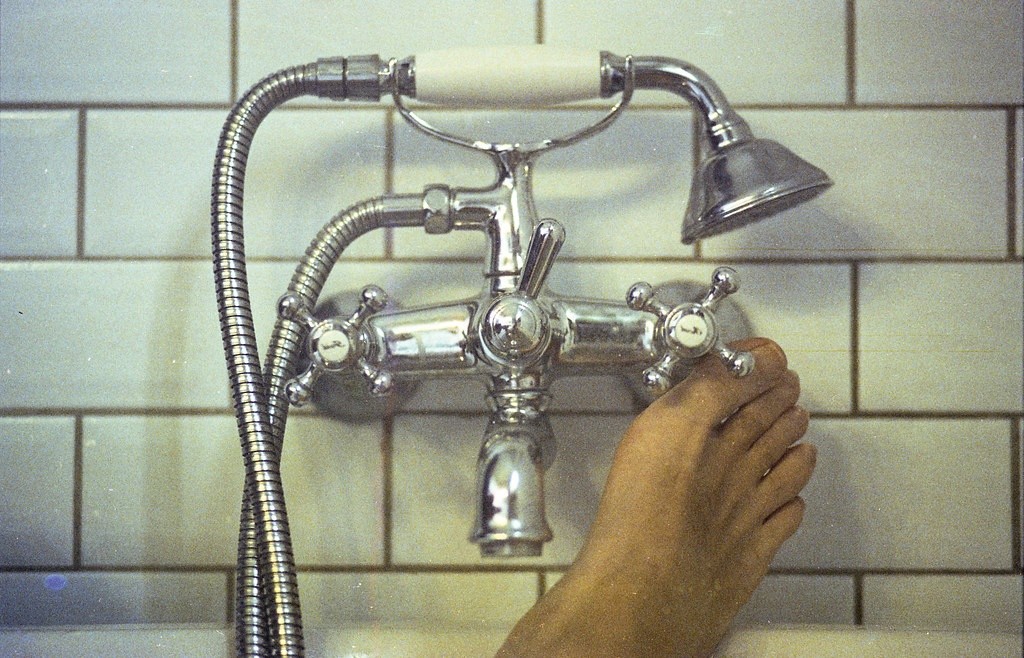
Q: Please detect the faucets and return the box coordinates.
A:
[459,384,564,562]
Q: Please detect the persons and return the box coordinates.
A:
[493,338,817,658]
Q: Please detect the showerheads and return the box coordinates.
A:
[674,95,834,250]
